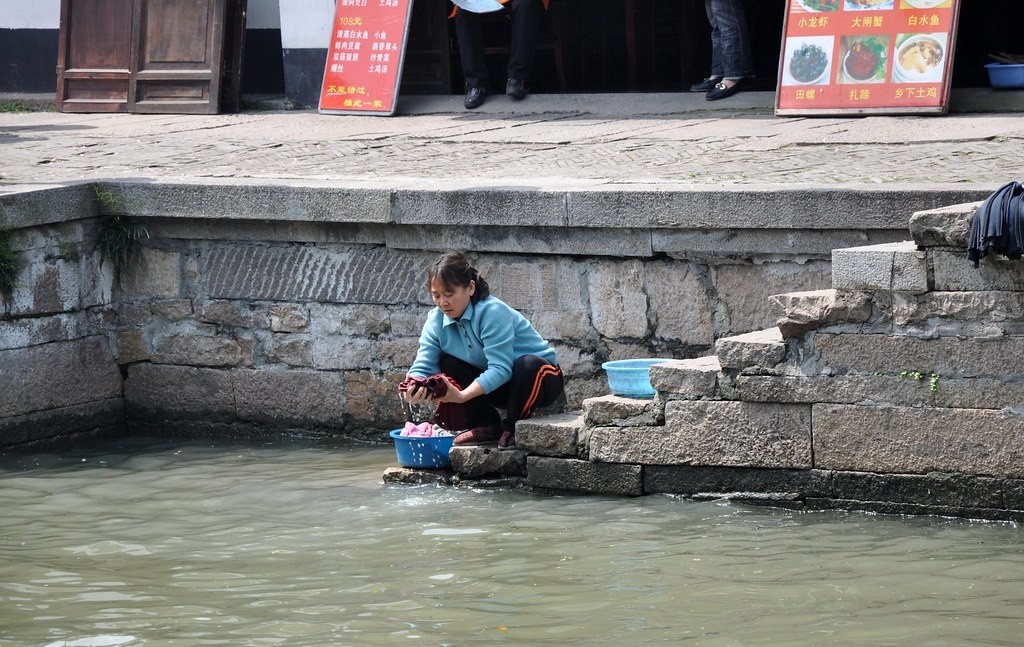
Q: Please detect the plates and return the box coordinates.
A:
[905,0,946,9]
[797,0,821,12]
[894,68,911,82]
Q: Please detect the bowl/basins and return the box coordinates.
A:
[390,430,456,469]
[843,49,886,82]
[788,54,829,84]
[984,63,1024,88]
[601,358,667,397]
[894,34,944,82]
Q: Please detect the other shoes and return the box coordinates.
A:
[452,425,499,446]
[506,77,527,100]
[464,87,488,108]
[705,80,744,101]
[690,76,723,91]
[497,431,517,450]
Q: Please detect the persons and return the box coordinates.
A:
[448,0,545,109]
[403,251,564,451]
[690,0,757,100]
